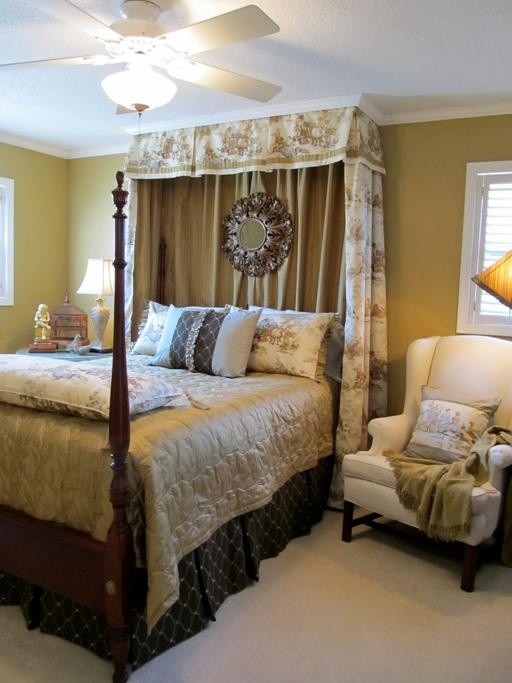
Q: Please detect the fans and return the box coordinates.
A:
[0,0,282,103]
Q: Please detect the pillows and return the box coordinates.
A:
[131,300,345,386]
[403,385,502,463]
[0,354,183,420]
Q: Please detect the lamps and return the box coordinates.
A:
[76,259,115,354]
[102,55,178,117]
[471,249,512,309]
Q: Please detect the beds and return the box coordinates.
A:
[0,108,391,683]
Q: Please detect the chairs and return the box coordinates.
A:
[341,335,512,592]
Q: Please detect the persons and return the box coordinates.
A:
[34,303,52,344]
